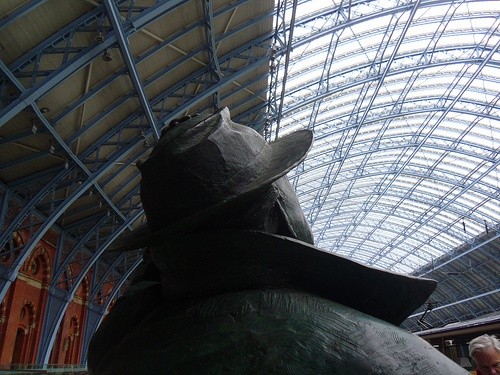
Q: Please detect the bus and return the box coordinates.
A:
[413,313,500,368]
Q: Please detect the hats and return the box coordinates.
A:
[107,106,315,254]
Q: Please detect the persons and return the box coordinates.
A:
[468,333,500,375]
[87,107,473,375]
